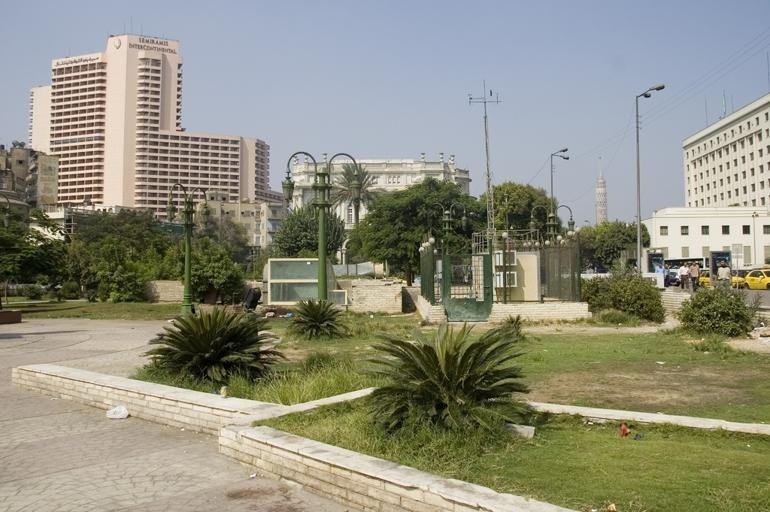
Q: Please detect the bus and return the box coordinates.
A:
[663,255,710,286]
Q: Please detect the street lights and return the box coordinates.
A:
[281,151,364,311]
[527,203,574,254]
[750,211,760,264]
[547,146,571,239]
[1,192,12,230]
[631,81,666,277]
[166,182,209,317]
[425,201,468,303]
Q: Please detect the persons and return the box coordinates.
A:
[717,259,731,286]
[690,263,697,288]
[695,260,700,285]
[678,262,690,291]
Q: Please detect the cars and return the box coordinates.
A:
[589,264,614,275]
[699,266,769,292]
